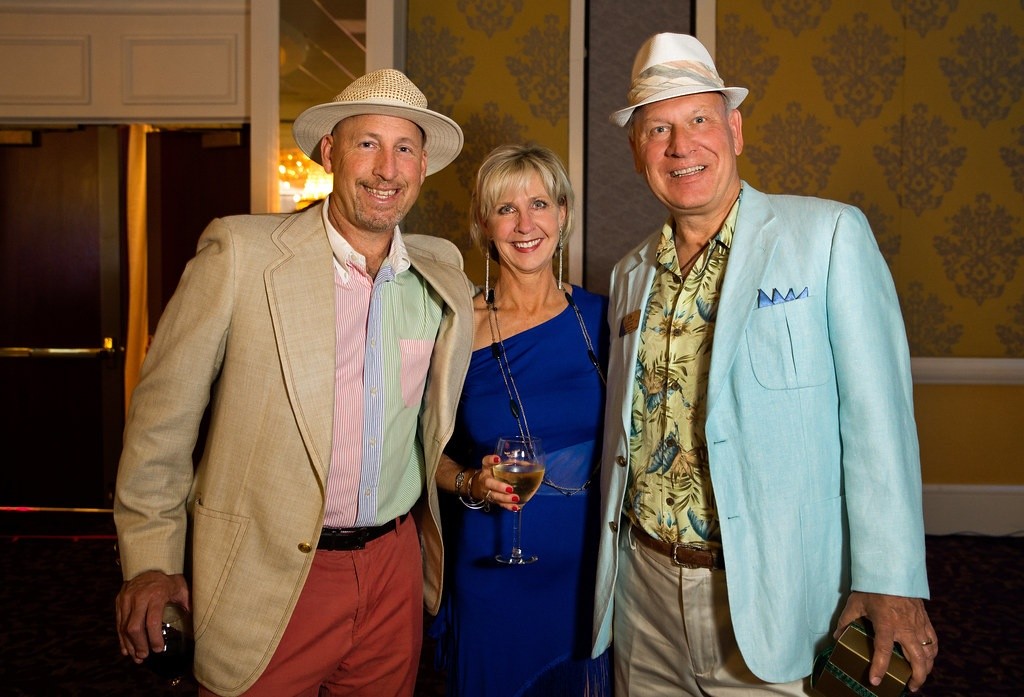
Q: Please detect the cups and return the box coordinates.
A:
[143,604,190,697]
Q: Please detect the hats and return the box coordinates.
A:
[609,31,749,130]
[292,69,464,178]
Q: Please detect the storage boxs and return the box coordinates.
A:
[811,617,912,697]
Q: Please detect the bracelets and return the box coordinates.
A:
[455,467,488,513]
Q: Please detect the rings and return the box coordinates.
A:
[919,639,933,647]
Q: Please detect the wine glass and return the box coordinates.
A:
[492,436,545,565]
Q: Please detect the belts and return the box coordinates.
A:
[623,514,726,572]
[315,512,411,551]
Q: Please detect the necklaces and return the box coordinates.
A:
[673,227,718,274]
[487,280,607,496]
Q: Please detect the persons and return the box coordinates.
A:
[589,31,937,697]
[437,143,611,696]
[114,69,485,697]
